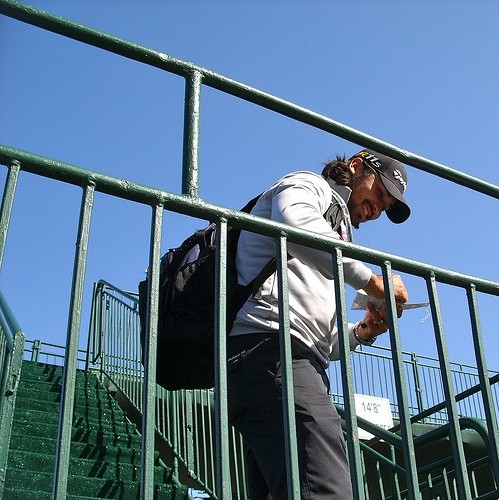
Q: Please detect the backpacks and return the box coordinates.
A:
[138,188,345,392]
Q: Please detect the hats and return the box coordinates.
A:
[348,149,411,224]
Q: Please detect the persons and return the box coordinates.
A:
[228,149,412,500]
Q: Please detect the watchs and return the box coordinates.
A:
[355,321,377,346]
